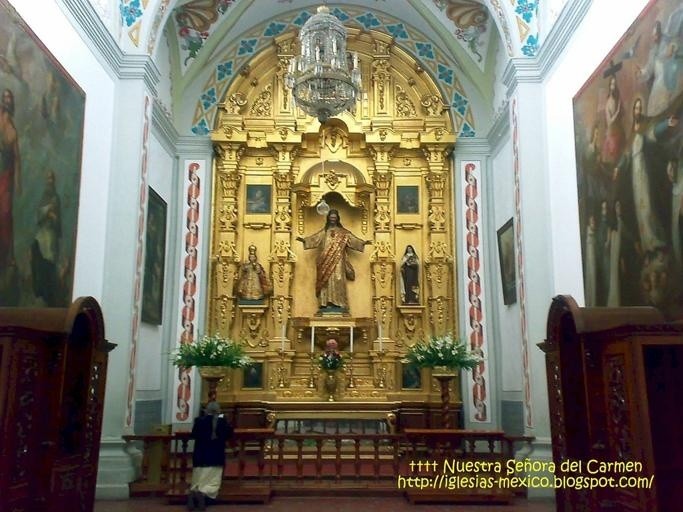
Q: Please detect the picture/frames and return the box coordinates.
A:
[141,184,167,325]
[496,217,517,306]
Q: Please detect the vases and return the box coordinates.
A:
[432,365,456,376]
[199,366,227,379]
[325,370,338,401]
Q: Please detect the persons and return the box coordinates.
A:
[27,169,66,307]
[295,208,371,319]
[183,401,239,511]
[235,240,267,300]
[399,245,421,308]
[575,4,682,306]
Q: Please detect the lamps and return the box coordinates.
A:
[285,0,364,122]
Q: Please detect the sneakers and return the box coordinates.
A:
[187,490,205,512]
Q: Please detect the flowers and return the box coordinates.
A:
[407,334,484,369]
[165,333,254,369]
[317,340,346,369]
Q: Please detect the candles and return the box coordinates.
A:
[349,325,353,352]
[281,325,286,351]
[311,326,315,355]
[378,324,383,350]
